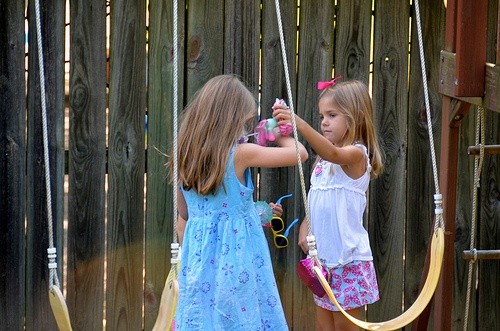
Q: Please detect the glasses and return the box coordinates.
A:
[270,193,301,249]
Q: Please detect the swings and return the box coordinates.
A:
[273,0,447,330]
[32,0,183,331]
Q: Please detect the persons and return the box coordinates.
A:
[151,202,282,331]
[175,74,308,330]
[272,78,383,330]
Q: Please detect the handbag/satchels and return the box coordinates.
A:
[299,258,331,299]
